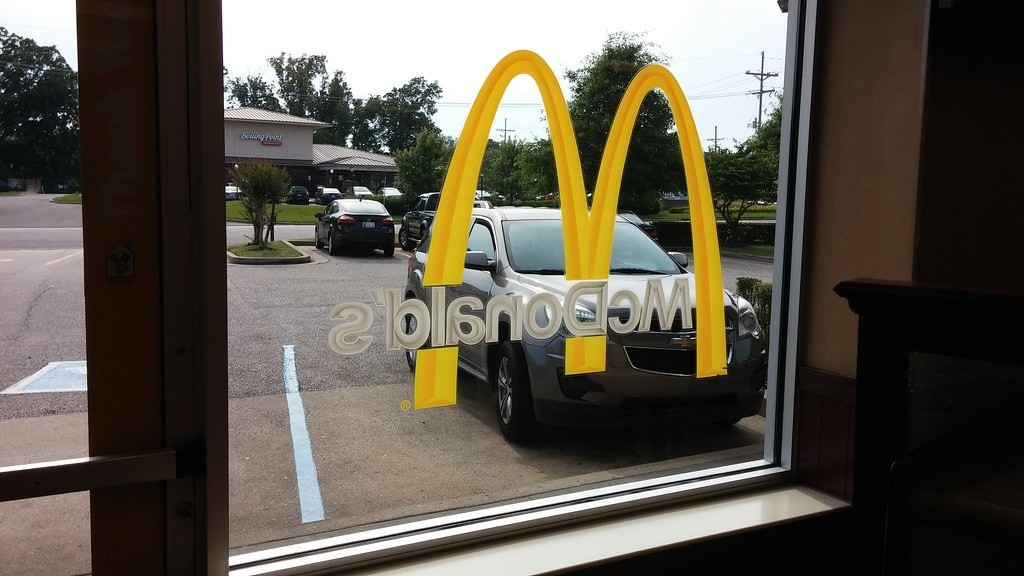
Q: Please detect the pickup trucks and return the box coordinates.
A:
[397,192,439,253]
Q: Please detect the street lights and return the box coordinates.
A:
[234,164,240,200]
[480,174,484,200]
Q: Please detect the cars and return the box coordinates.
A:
[475,190,492,200]
[314,197,396,258]
[346,185,374,196]
[403,207,766,446]
[376,187,403,200]
[225,186,244,200]
[314,187,344,204]
[285,186,309,205]
